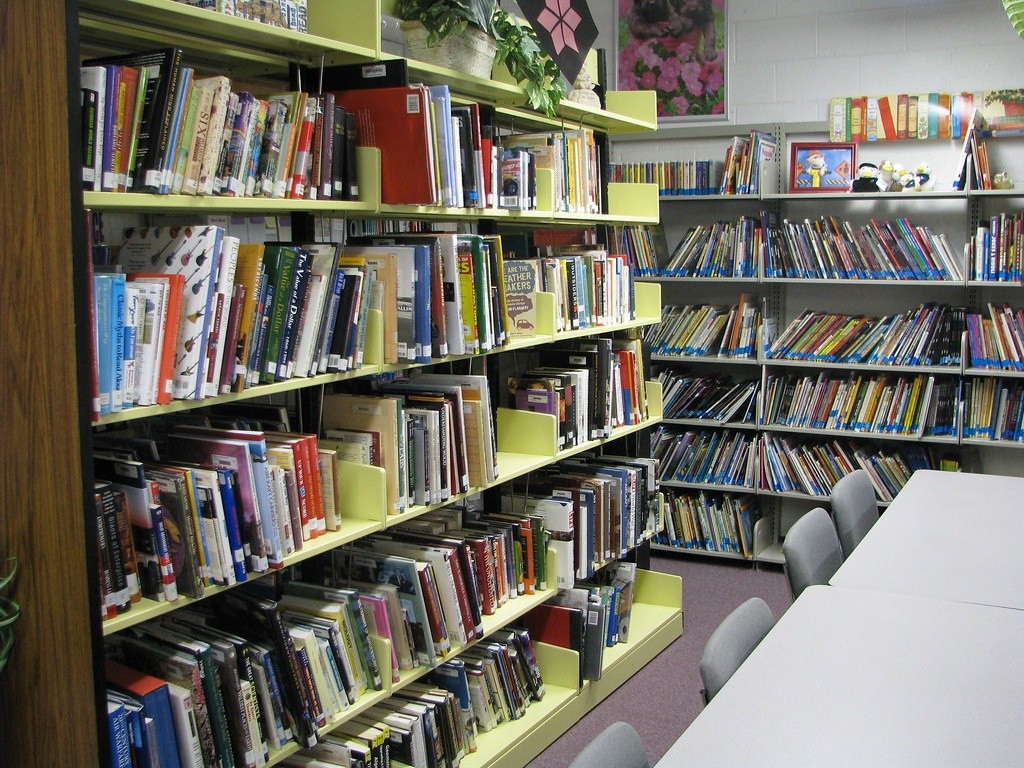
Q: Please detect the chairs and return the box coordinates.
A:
[566,721,651,767]
[700,596,775,709]
[829,467,880,559]
[781,507,846,604]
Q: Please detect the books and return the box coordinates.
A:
[86,333,660,768]
[953,107,1024,190]
[641,300,1024,372]
[87,220,636,416]
[646,427,962,503]
[652,487,757,558]
[607,128,760,196]
[608,205,1024,280]
[644,369,1024,444]
[76,1,605,213]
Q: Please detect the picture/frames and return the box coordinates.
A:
[789,141,860,194]
[615,0,728,120]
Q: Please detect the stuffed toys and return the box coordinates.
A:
[851,162,934,192]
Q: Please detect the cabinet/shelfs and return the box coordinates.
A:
[0,0,685,767]
[607,120,1024,572]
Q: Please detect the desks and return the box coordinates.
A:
[655,583,1024,767]
[827,469,1024,610]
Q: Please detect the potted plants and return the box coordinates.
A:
[395,0,569,120]
